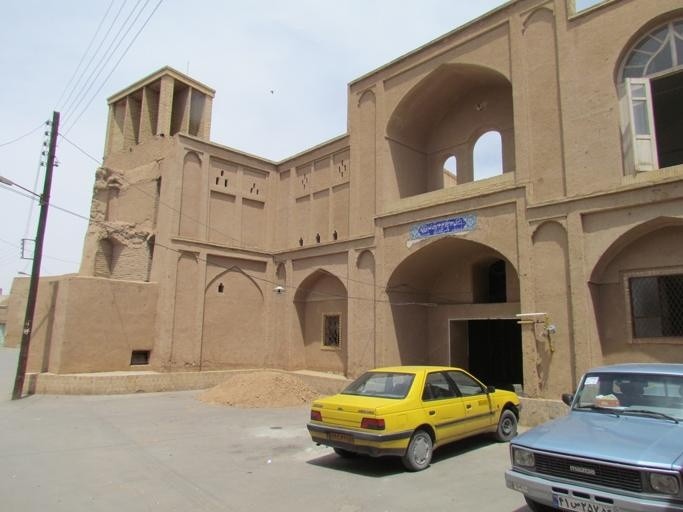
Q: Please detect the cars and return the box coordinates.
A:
[504,364,682,511]
[305,364,522,471]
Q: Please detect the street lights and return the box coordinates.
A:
[0,176,50,400]
